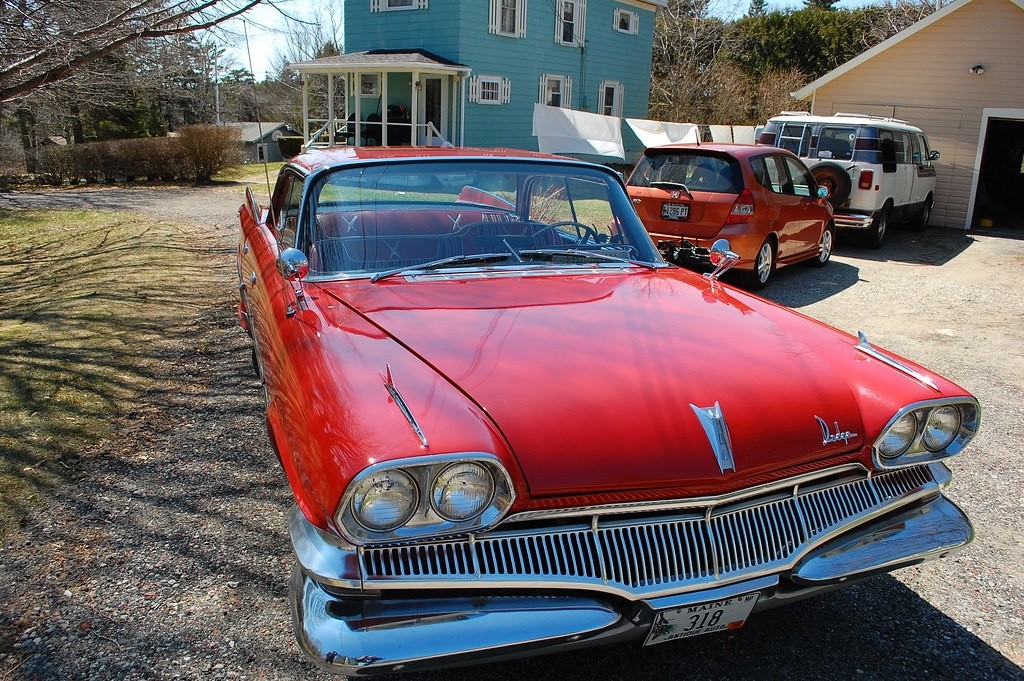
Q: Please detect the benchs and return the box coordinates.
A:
[287,211,563,276]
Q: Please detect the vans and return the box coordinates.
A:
[756,110,941,250]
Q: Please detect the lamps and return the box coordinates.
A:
[968,65,985,75]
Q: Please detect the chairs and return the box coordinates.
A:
[829,139,850,151]
[687,163,716,188]
[334,113,362,146]
[714,166,732,190]
[361,113,380,146]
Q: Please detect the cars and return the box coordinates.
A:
[236,146,983,678]
[612,141,837,289]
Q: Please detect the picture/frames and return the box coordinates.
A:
[354,73,382,98]
